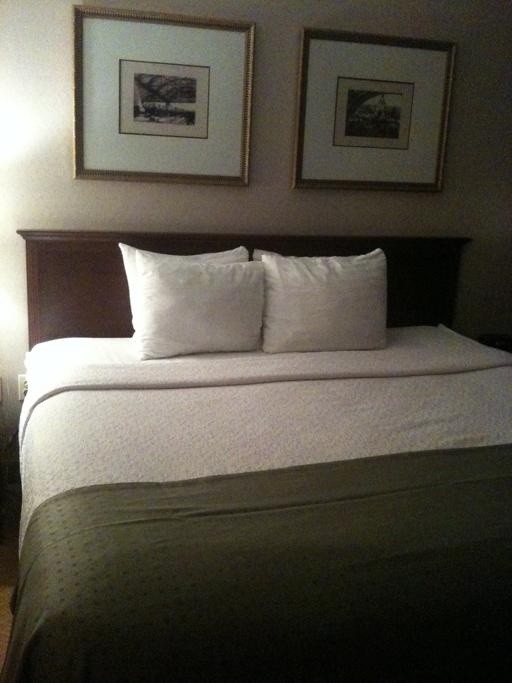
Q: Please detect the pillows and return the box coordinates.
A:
[119,241,391,359]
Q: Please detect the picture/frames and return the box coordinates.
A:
[291,26,456,192]
[73,3,256,188]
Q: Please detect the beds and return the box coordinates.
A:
[18,227,512,683]
